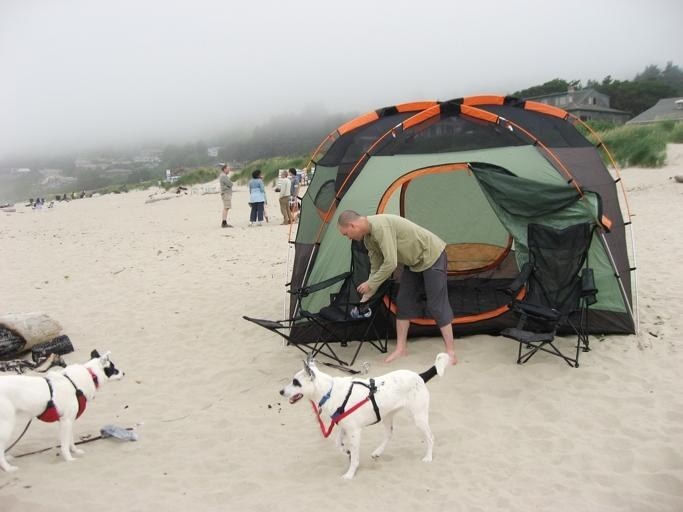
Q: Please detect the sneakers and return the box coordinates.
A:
[279,218,295,225]
[248,216,269,227]
[222,220,234,227]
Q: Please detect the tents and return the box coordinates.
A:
[286,95,636,345]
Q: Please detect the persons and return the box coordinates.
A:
[336,210,458,369]
[219,165,232,228]
[248,169,266,227]
[258,176,267,220]
[288,167,300,223]
[279,170,292,225]
[71,192,74,199]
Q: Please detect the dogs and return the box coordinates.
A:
[276,351,451,482]
[0,349,125,472]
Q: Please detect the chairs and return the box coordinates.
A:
[241,239,395,366]
[497,223,598,368]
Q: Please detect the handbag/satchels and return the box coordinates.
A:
[290,202,301,212]
